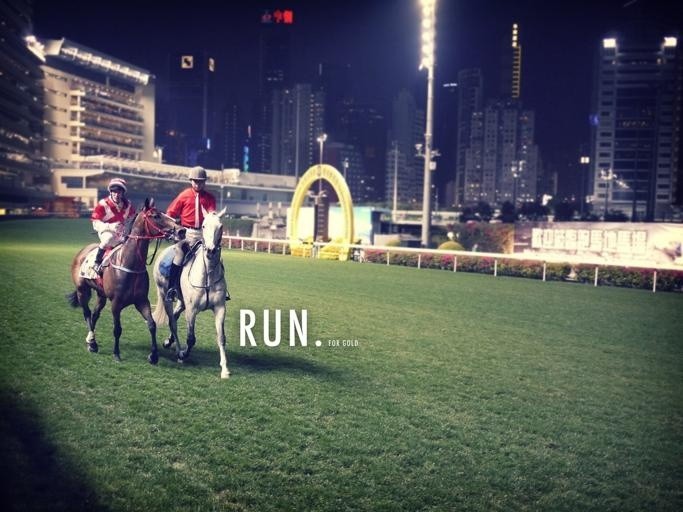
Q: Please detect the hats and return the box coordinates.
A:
[108,179,127,193]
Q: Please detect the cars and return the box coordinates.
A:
[573,210,649,221]
[460,201,492,222]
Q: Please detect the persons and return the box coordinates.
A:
[92,177,135,276]
[167,166,232,300]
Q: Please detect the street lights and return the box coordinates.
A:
[417,2,440,242]
[599,167,613,221]
[511,159,525,216]
[580,156,589,220]
[314,132,328,200]
[342,158,349,180]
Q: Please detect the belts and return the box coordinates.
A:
[183,225,201,230]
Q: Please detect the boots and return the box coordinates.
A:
[165,261,182,301]
[96,247,105,262]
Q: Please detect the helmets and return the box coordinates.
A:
[189,166,208,181]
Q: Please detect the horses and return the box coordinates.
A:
[143,204,232,379]
[64,196,187,366]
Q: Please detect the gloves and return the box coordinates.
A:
[108,223,120,232]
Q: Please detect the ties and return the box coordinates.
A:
[195,193,199,228]
